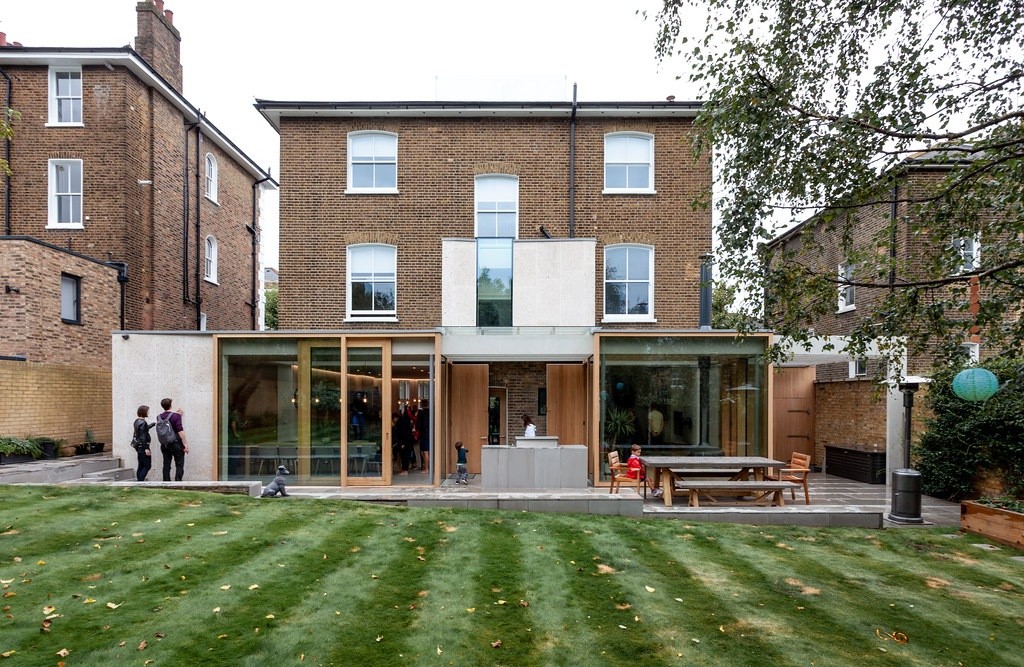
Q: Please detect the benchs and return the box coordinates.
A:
[660,469,801,508]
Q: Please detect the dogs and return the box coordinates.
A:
[260,464,290,498]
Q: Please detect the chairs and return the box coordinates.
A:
[257,442,397,476]
[763,451,812,505]
[607,451,647,500]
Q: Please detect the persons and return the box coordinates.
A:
[521,414,536,437]
[454,442,468,485]
[156,398,189,481]
[647,402,664,444]
[392,399,430,476]
[229,404,243,474]
[626,444,663,496]
[133,406,157,481]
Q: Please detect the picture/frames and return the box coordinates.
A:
[538,387,546,416]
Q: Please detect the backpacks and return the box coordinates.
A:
[156,412,177,448]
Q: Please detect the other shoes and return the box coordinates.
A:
[654,489,663,496]
[456,480,460,484]
[462,479,468,484]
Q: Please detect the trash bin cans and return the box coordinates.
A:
[889,469,924,523]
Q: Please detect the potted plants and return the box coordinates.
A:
[0,436,64,465]
[604,406,637,475]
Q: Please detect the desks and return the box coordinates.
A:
[641,456,786,507]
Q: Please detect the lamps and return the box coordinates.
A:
[879,376,941,469]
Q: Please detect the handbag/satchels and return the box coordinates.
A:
[131,438,141,448]
